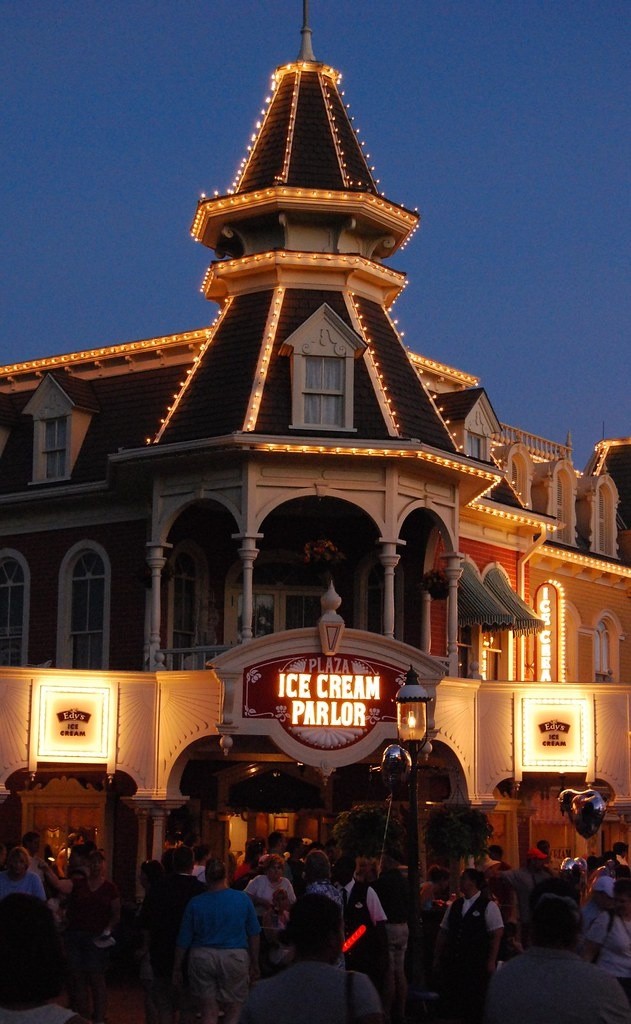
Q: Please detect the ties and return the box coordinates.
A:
[340,888,347,906]
[532,875,537,886]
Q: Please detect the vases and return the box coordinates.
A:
[425,584,448,601]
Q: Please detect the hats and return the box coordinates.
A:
[90,849,108,860]
[593,876,616,897]
[526,848,547,859]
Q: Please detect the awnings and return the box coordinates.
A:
[483,569,544,635]
[457,563,515,631]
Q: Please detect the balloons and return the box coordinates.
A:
[558,788,609,838]
[380,744,413,792]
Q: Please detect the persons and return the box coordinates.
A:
[1,832,631,1024]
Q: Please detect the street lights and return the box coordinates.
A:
[392,665,435,989]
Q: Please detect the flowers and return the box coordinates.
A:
[303,537,343,565]
[417,567,449,589]
